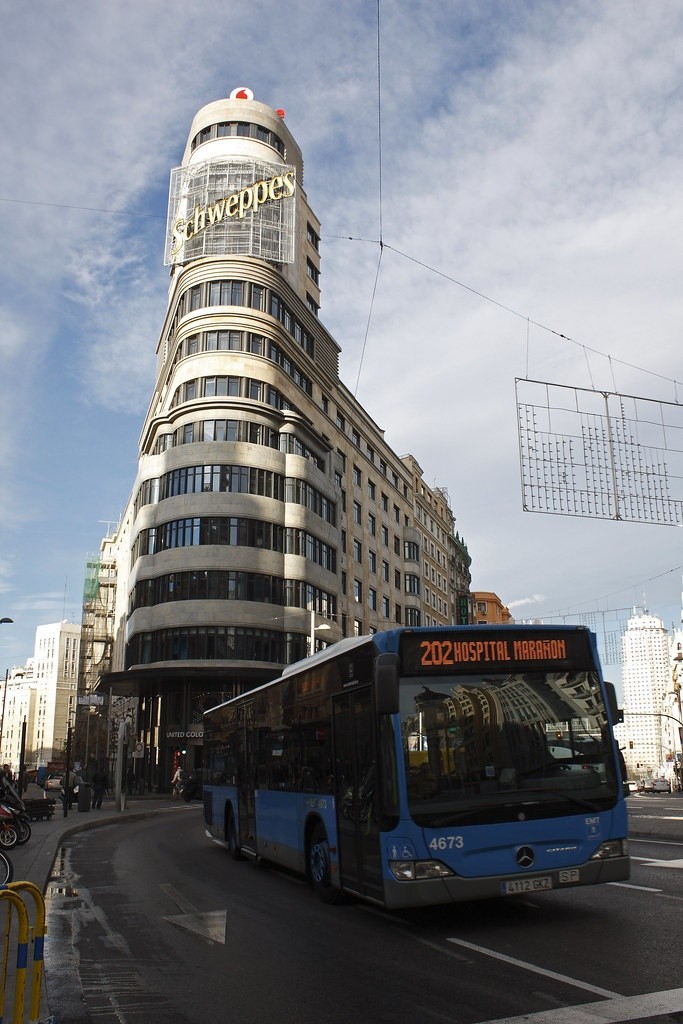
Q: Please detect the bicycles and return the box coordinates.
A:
[168,781,183,802]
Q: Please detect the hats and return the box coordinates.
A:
[177,765,182,768]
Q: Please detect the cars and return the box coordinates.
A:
[623,780,638,791]
[546,740,630,798]
[15,769,38,783]
[645,784,652,793]
[183,770,224,802]
[44,774,64,791]
[408,735,466,774]
[652,778,671,794]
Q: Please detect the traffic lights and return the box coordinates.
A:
[618,710,624,723]
[629,741,633,749]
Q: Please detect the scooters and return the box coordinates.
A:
[341,767,377,823]
[0,777,32,849]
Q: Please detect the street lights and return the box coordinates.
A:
[0,668,26,745]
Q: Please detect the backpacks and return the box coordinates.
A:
[179,771,186,780]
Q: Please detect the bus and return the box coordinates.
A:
[201,625,631,909]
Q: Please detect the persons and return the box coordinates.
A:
[92,768,106,809]
[127,768,134,794]
[170,766,186,793]
[1,764,12,791]
[22,766,30,795]
[60,766,77,809]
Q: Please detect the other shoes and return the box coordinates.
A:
[179,789,183,794]
